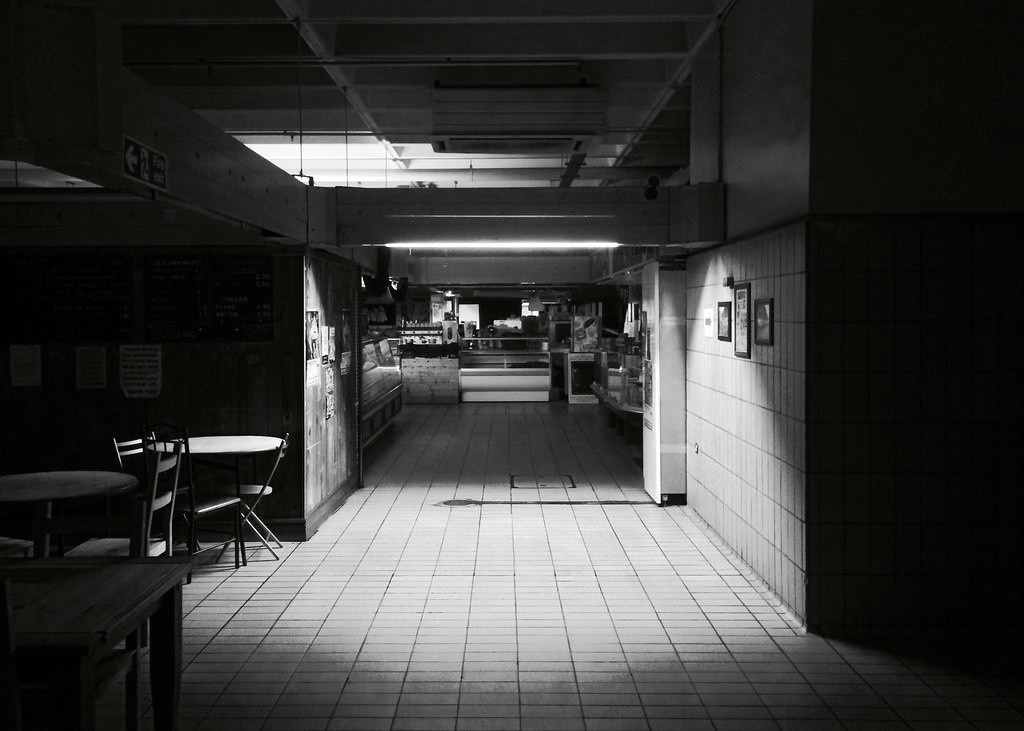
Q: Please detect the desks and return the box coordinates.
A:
[2,556,192,731]
[147,435,287,587]
[0,471,138,558]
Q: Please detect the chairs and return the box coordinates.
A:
[0,422,291,730]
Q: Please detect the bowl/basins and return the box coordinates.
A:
[428,339,437,343]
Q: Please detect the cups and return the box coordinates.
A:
[422,340,427,343]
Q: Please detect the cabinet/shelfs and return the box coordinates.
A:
[458,336,553,402]
[359,334,403,449]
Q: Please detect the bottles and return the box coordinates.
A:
[407,321,442,327]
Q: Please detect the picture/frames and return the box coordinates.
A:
[717,301,732,341]
[734,283,751,358]
[752,298,774,346]
